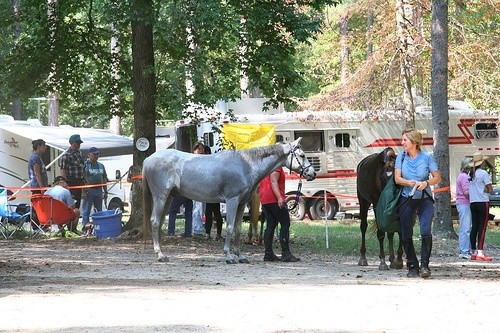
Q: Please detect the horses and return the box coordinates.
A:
[140,136,317,264]
[356,148,405,270]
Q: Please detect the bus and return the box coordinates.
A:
[195,106,500,224]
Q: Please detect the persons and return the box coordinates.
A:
[29,135,109,237]
[395,129,494,277]
[167,136,301,262]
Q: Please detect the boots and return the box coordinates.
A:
[401,238,420,278]
[420,234,433,277]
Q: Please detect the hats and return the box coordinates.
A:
[53,176,69,184]
[88,147,100,154]
[468,152,488,167]
[69,134,83,144]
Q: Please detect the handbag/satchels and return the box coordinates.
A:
[374,174,416,233]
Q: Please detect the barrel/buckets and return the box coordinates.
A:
[91,210,122,238]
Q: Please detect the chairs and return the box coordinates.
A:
[0,187,76,239]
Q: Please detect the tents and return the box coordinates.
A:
[0,124,137,216]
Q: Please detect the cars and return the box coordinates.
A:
[489,187,500,207]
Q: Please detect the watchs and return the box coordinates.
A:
[426,181,430,186]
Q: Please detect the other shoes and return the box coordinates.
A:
[476,250,492,261]
[470,249,477,260]
[459,252,471,259]
[216,234,223,241]
[263,249,280,261]
[281,250,301,262]
[203,232,210,239]
